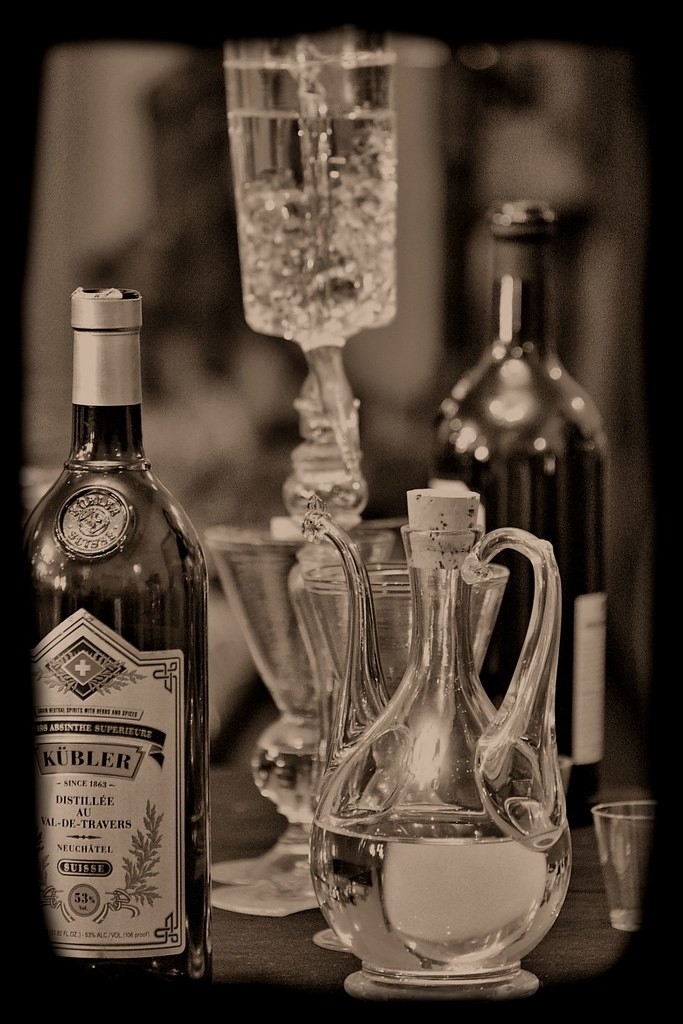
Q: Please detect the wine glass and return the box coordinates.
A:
[204,528,359,915]
[303,561,509,946]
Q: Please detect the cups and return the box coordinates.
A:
[591,800,653,930]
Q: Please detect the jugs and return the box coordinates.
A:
[301,490,575,999]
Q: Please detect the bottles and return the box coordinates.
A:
[428,201,605,829]
[25,290,220,989]
